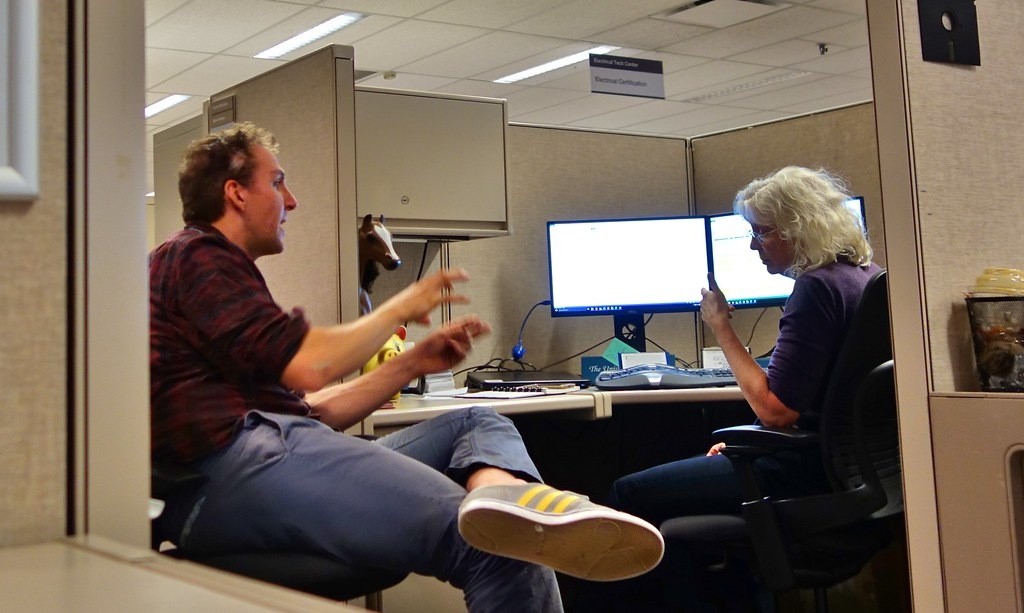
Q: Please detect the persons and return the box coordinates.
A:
[148,122,666,613]
[602,164,879,613]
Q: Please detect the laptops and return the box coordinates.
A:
[468,370,590,390]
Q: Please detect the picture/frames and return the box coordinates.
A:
[0,0,40,201]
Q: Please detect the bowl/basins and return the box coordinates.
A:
[970,268,1024,294]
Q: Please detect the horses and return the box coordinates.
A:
[357,213,402,294]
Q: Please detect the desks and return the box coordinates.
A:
[928,386,1024,613]
[363,369,751,438]
[0,532,365,613]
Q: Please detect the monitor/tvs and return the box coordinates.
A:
[546,195,869,353]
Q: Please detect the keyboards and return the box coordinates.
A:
[595,363,740,390]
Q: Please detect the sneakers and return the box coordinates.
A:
[456,484,664,581]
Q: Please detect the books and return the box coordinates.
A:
[455,382,581,400]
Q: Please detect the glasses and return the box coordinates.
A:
[747,227,780,241]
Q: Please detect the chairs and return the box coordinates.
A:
[698,270,905,613]
[150,436,410,612]
